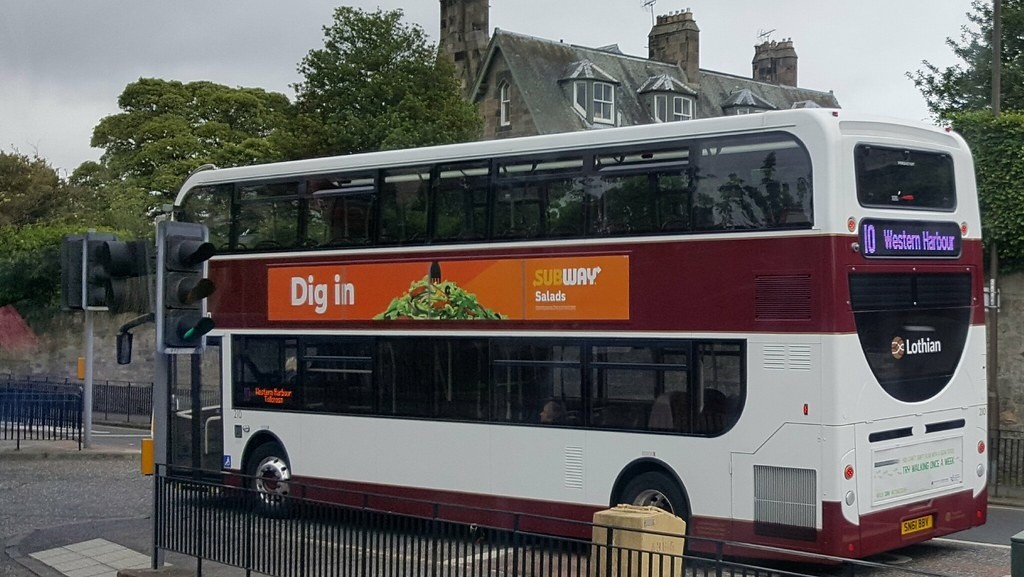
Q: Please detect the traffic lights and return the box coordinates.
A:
[96,239,154,315]
[157,221,217,353]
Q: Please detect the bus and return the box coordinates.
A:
[116,112,990,565]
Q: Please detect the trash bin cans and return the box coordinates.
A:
[590,503,686,577]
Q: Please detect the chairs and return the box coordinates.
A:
[254,240,282,248]
[661,219,688,232]
[457,230,486,240]
[593,405,624,426]
[363,234,400,246]
[648,390,689,431]
[329,236,357,247]
[549,224,578,237]
[501,228,534,240]
[694,388,729,432]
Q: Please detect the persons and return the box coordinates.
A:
[284,358,298,380]
[539,402,568,424]
